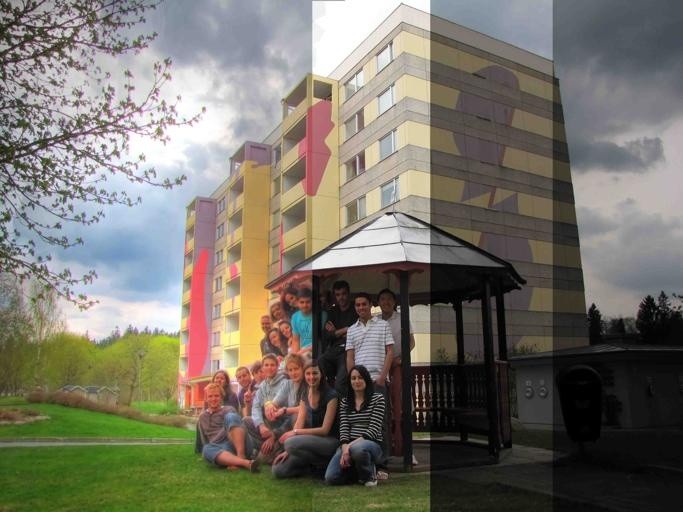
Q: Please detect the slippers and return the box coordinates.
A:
[248,459,261,473]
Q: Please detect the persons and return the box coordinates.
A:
[193,278,421,489]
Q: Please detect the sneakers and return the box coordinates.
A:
[364,476,379,487]
